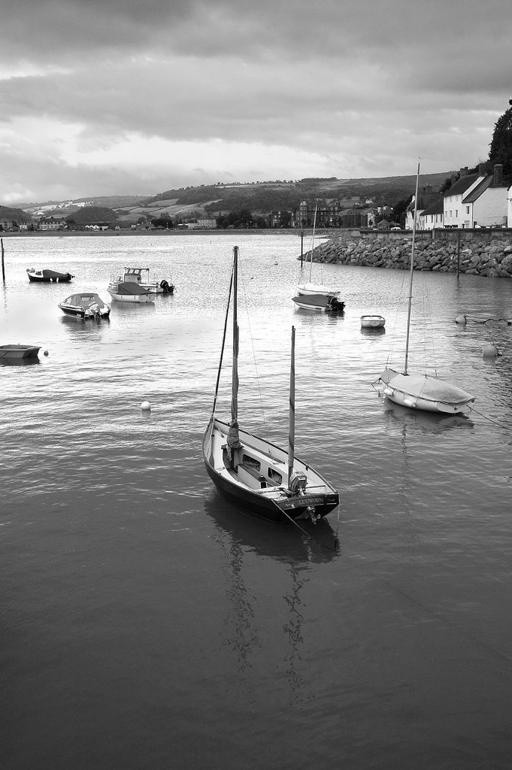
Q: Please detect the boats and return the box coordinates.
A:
[0,342,40,360]
[360,314,385,327]
[58,292,111,321]
[26,267,71,284]
[139,281,174,293]
[109,266,156,305]
[293,306,345,319]
[0,357,40,367]
[360,326,385,337]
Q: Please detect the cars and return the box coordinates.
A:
[390,227,401,230]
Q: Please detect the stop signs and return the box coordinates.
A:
[198,241,340,530]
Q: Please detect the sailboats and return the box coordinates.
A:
[200,485,342,676]
[289,197,340,318]
[382,397,474,472]
[377,159,475,425]
